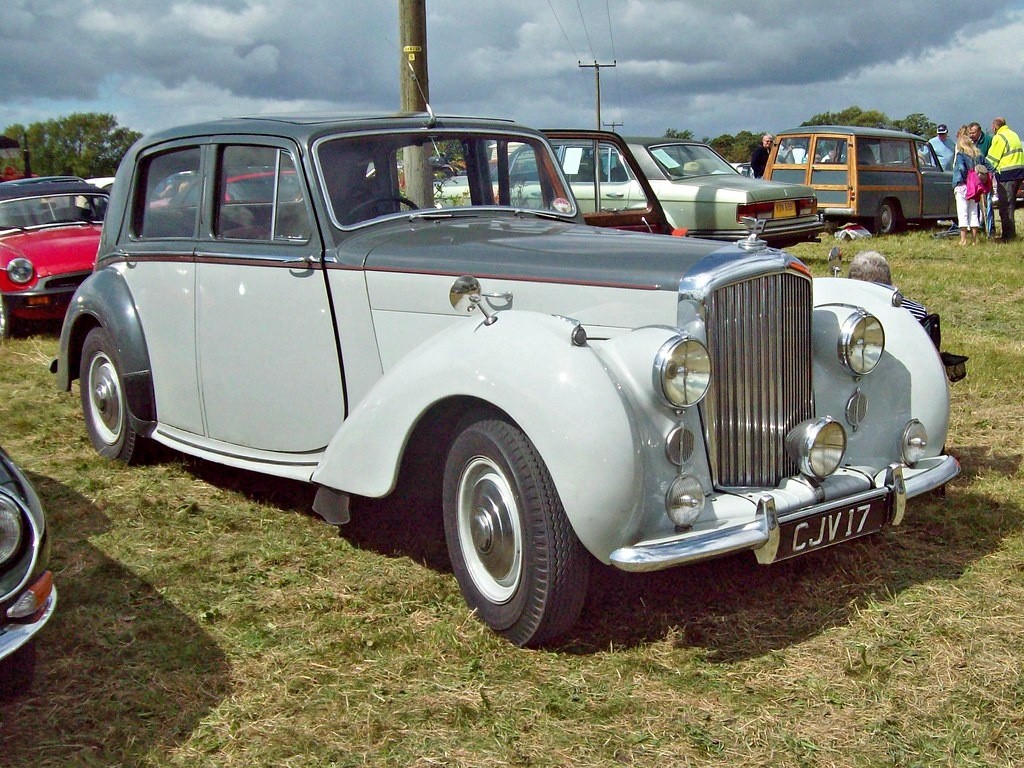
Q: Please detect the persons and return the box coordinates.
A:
[917,124,956,171]
[953,122,996,246]
[150,175,192,208]
[985,117,1024,244]
[748,133,773,179]
[847,251,931,336]
[776,138,845,164]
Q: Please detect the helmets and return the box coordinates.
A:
[937,124,947,134]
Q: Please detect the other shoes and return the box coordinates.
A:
[959,240,967,246]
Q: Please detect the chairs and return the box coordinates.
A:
[918,313,970,383]
[586,158,607,180]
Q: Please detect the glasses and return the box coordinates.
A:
[765,140,772,143]
[939,133,945,135]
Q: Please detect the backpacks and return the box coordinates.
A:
[975,164,988,185]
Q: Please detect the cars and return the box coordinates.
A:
[0,445,61,666]
[0,175,122,341]
[47,108,963,649]
[74,124,1024,244]
